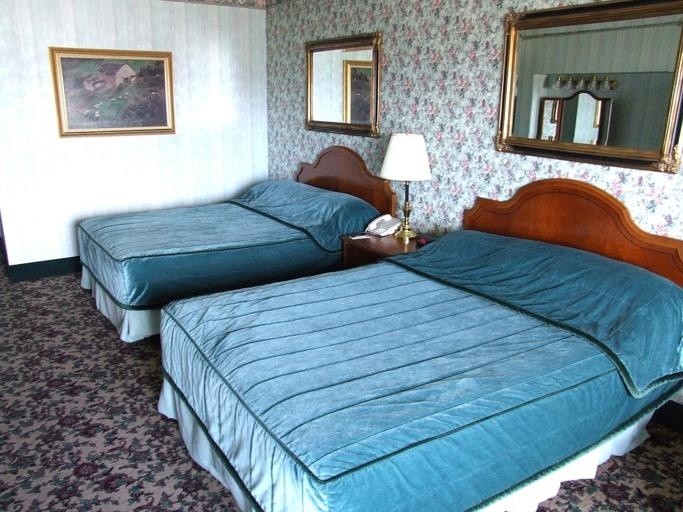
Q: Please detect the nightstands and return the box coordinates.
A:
[341,231,438,269]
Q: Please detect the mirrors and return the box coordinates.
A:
[304,32,380,137]
[494,0,683,174]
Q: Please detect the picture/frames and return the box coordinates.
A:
[531,65,622,148]
[342,58,372,124]
[48,46,176,138]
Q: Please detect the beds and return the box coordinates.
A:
[162,176,683,512]
[78,146,398,345]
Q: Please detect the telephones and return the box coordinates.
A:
[366,214,402,236]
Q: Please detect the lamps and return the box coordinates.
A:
[381,133,437,240]
